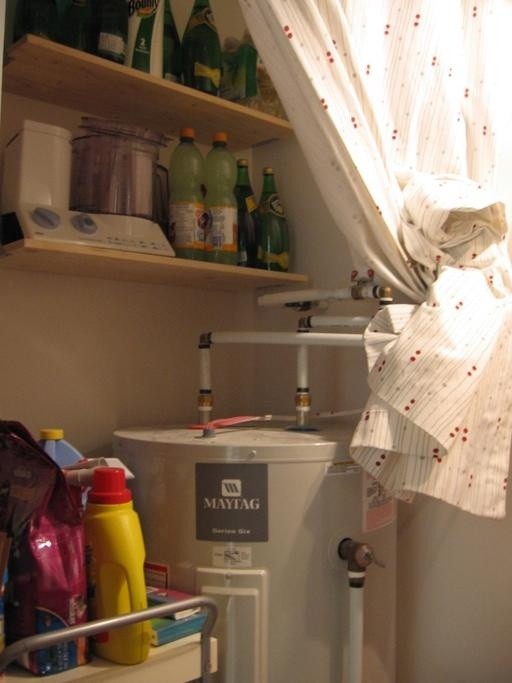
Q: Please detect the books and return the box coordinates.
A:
[145,586,210,647]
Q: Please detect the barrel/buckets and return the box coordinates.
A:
[38,427,86,470]
[83,465,152,666]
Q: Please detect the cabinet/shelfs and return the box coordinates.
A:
[3,27,310,289]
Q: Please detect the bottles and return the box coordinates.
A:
[167,124,291,270]
[12,0,262,100]
